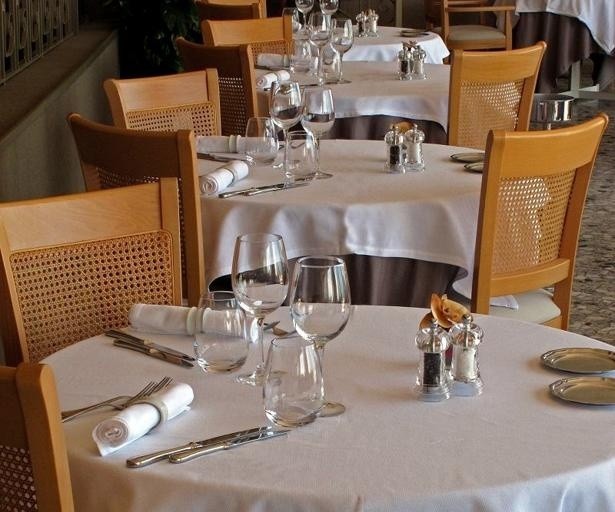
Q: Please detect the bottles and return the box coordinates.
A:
[355,9,426,174]
[410,319,451,401]
[448,313,486,397]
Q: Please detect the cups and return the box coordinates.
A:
[244,116,280,165]
[195,291,248,376]
[264,336,325,428]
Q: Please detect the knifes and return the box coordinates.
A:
[196,153,247,162]
[247,181,310,196]
[112,338,195,373]
[126,426,269,468]
[105,328,196,369]
[169,429,291,464]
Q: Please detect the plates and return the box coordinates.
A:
[450,152,490,162]
[549,375,615,405]
[464,162,484,173]
[540,348,615,374]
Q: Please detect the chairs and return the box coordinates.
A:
[170,36,260,137]
[2,177,183,359]
[0,361,76,512]
[447,40,547,152]
[450,112,609,330]
[195,0,266,19]
[68,113,208,308]
[425,0,515,64]
[103,67,223,136]
[200,16,293,66]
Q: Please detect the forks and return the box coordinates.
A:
[61,375,176,422]
[61,380,159,423]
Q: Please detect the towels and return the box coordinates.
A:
[128,304,245,338]
[256,70,290,88]
[195,135,276,155]
[256,54,289,67]
[92,381,195,456]
[198,159,249,196]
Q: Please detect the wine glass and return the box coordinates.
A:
[268,0,352,179]
[288,256,351,416]
[231,230,288,387]
